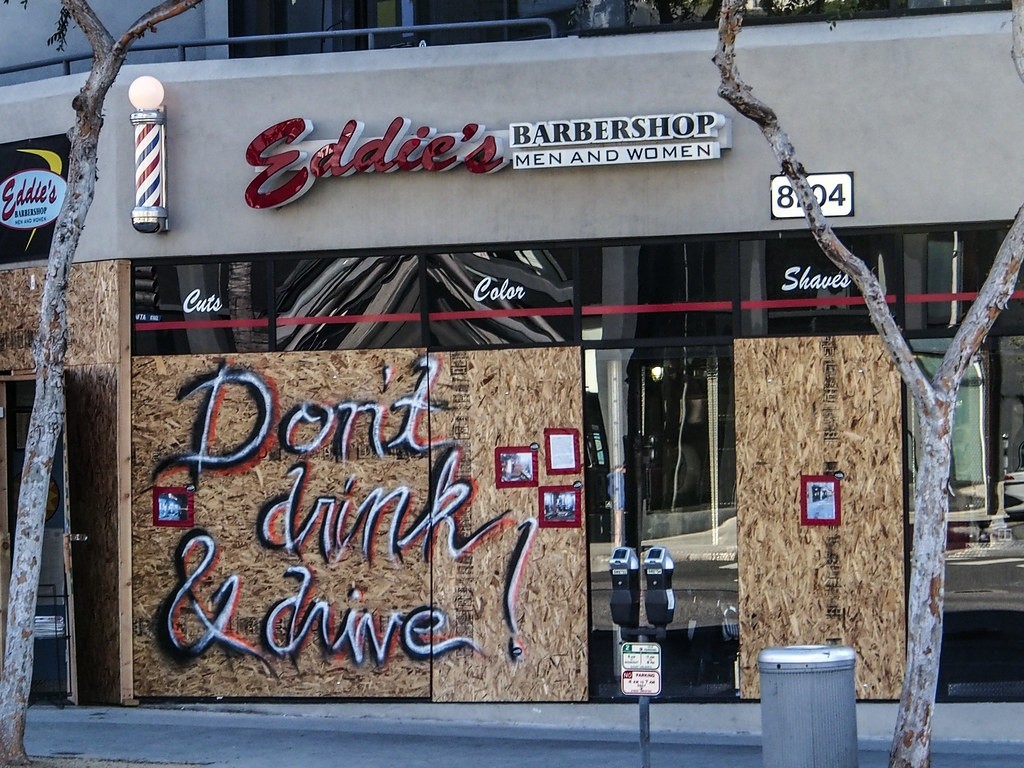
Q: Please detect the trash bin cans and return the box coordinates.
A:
[758,641,860,768]
